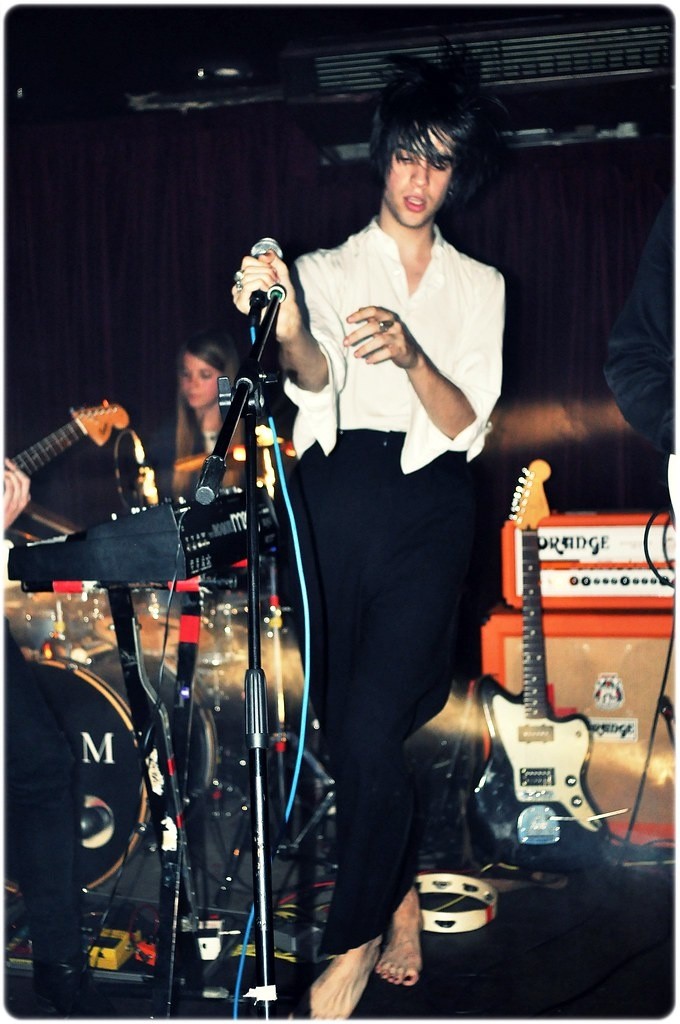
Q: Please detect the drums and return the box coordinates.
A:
[7,599,102,650]
[25,633,216,895]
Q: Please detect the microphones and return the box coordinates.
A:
[78,806,111,838]
[128,432,158,506]
[659,695,673,721]
[246,238,283,328]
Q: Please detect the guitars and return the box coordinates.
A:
[470,459,610,869]
[5,396,131,495]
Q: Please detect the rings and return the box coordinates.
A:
[378,321,389,332]
[234,270,245,282]
[236,280,243,291]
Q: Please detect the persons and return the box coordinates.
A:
[148,334,245,503]
[601,190,675,452]
[4,459,119,1017]
[231,73,506,1020]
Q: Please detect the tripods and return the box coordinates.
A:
[210,552,336,923]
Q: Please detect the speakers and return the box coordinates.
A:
[483,606,677,851]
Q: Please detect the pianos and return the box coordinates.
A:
[7,483,283,597]
